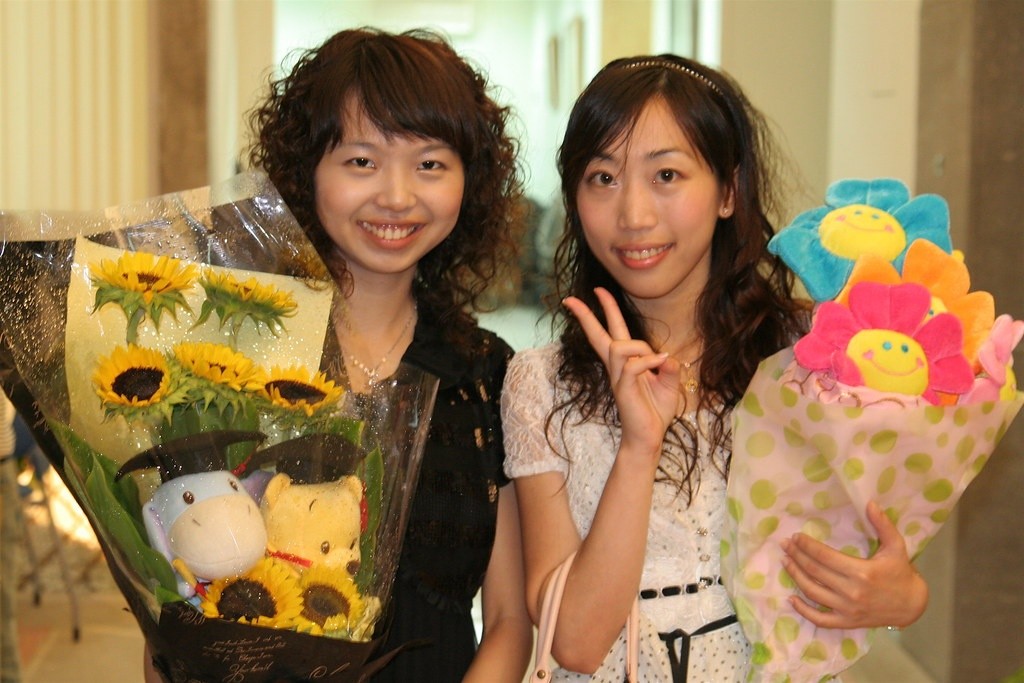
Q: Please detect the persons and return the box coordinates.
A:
[498,53,929,683]
[143,28,532,683]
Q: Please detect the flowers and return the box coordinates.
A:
[46,246,383,640]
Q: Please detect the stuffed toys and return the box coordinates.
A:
[114,430,269,613]
[766,180,1024,408]
[245,434,367,581]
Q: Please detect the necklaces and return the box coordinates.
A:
[343,300,417,389]
[680,354,704,393]
